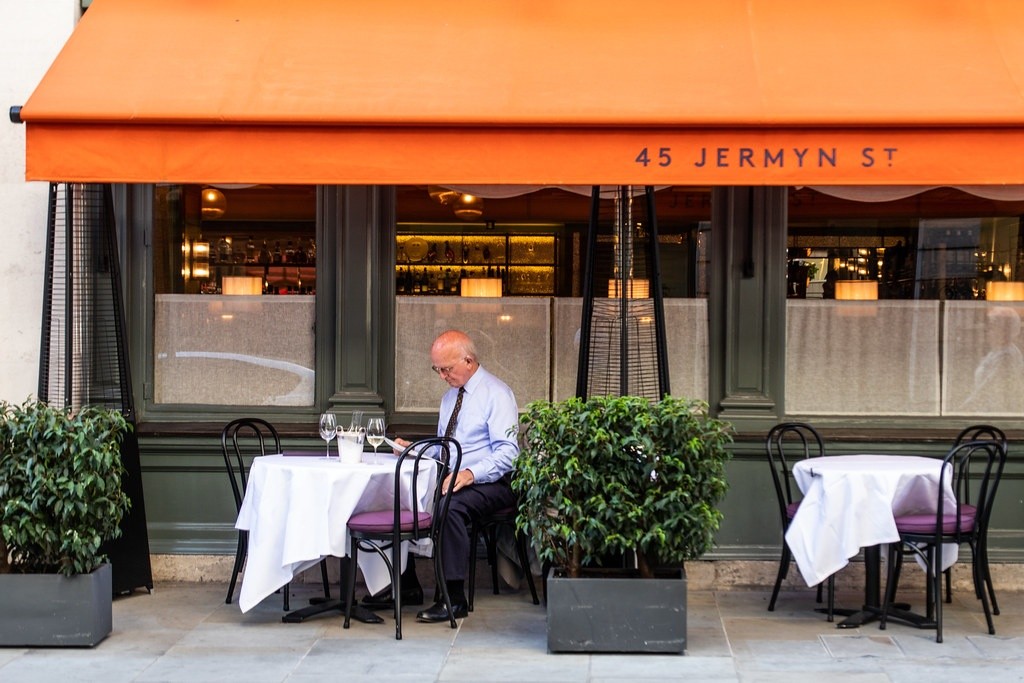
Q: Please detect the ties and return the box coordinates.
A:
[436,386,465,477]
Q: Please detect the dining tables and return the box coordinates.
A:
[793,455,959,629]
[234,452,437,630]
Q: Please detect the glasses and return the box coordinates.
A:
[431,359,464,375]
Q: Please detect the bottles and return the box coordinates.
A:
[199,235,506,297]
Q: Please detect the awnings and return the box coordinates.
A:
[9,0,1024,186]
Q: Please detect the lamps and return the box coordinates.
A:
[608,279,649,319]
[461,277,503,313]
[192,239,210,279]
[221,275,262,314]
[835,279,878,315]
[202,189,228,221]
[986,282,1024,321]
[428,185,483,221]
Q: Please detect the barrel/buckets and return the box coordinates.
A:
[336,425,366,463]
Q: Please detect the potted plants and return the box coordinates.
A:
[504,393,737,652]
[0,393,134,646]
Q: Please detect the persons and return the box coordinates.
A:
[381,331,520,622]
[823,270,839,299]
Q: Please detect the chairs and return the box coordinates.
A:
[767,421,1009,643]
[223,419,540,640]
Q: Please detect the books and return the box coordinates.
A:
[385,437,452,469]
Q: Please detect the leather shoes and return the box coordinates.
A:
[416,593,469,622]
[362,582,425,607]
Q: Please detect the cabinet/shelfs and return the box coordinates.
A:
[176,229,561,307]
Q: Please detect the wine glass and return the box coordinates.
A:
[319,414,337,460]
[366,419,385,463]
